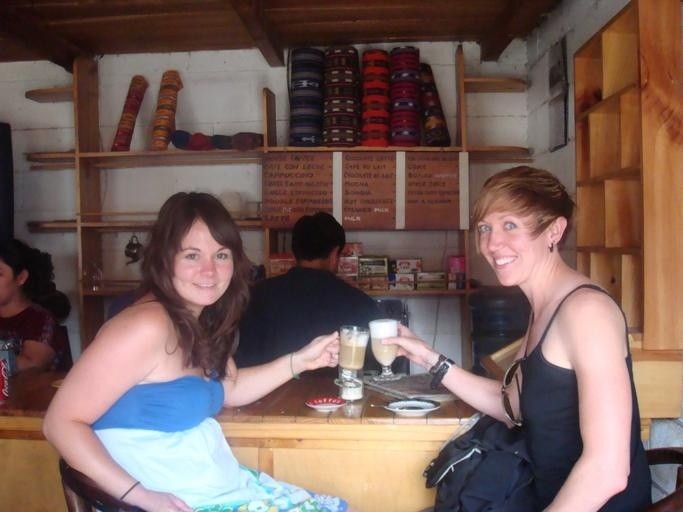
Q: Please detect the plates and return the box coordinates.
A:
[304,395,347,412]
[383,396,443,417]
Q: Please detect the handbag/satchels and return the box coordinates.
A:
[424,415,536,511]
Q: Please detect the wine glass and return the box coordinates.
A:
[368,318,403,382]
[333,324,369,390]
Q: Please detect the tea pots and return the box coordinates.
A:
[124,235,143,265]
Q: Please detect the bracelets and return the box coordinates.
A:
[119,480,140,501]
[430,358,456,389]
[428,353,447,376]
[289,350,300,380]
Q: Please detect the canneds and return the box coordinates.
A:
[0,358,9,401]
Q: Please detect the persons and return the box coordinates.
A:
[368,164,655,512]
[231,212,400,375]
[42,190,366,512]
[0,236,75,379]
